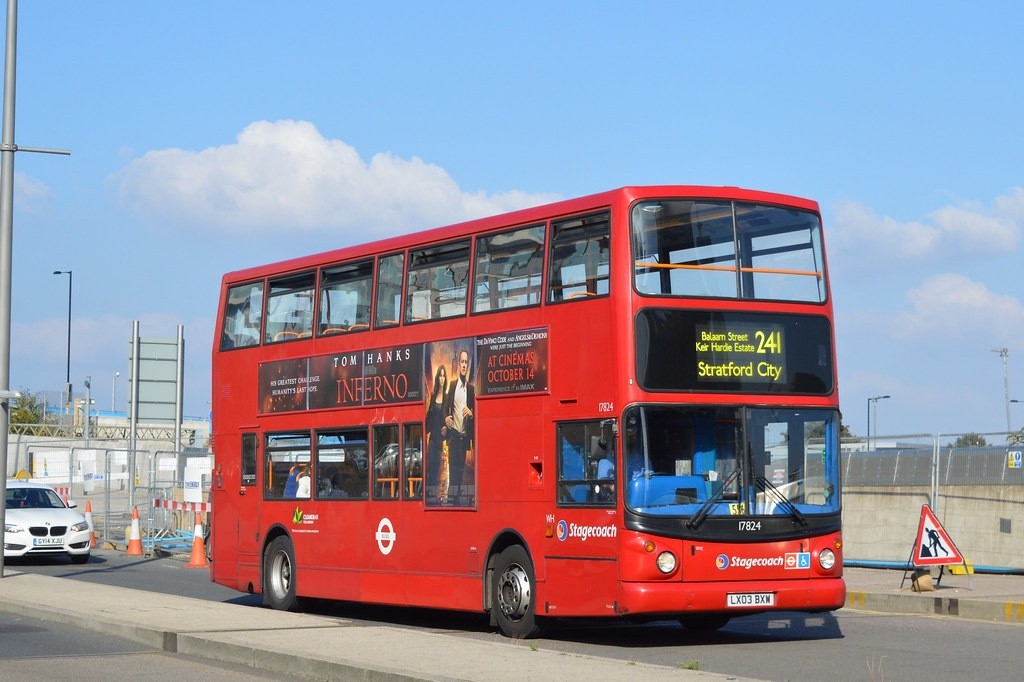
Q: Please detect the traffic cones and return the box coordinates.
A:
[84,501,97,548]
[127,506,144,557]
[183,512,211,569]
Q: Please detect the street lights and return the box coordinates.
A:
[866,395,891,451]
[54,267,74,407]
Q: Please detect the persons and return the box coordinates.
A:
[424,351,475,505]
[23,489,42,507]
[597,428,653,497]
[296,462,332,498]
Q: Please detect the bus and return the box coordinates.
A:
[209,185,848,641]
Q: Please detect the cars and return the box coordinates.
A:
[2,479,91,565]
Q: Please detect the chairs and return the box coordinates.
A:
[568,292,594,299]
[282,463,310,498]
[273,314,427,342]
